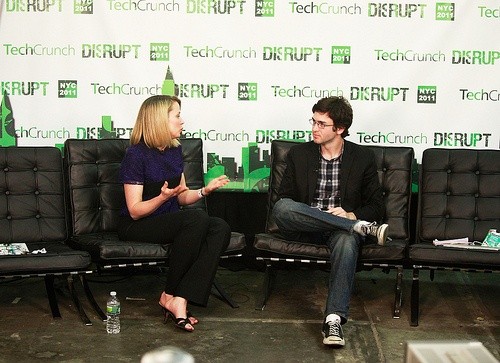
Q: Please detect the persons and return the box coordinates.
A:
[117,95,231,331]
[271,96,388,345]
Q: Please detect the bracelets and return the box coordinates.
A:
[198,189,204,198]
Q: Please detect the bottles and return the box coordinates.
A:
[106,291,120,334]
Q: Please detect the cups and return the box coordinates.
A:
[140,345,195,363]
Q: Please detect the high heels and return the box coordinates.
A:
[158,301,199,332]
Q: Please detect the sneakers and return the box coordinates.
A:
[360,220,389,246]
[321,319,346,346]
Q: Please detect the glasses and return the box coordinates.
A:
[309,118,334,129]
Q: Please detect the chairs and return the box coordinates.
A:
[410,148,500,326]
[0,146,94,327]
[253,140,414,318]
[64,137,246,321]
